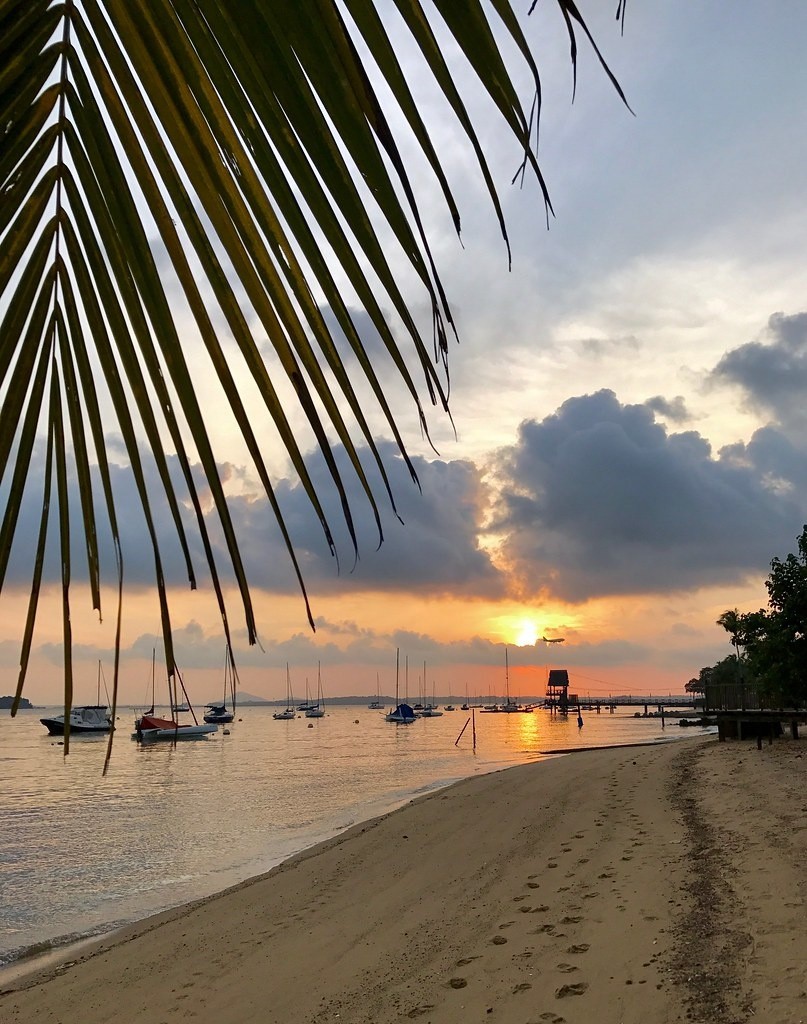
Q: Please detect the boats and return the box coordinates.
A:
[37,643,235,743]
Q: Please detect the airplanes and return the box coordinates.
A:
[542,636,565,644]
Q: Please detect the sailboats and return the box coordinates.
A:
[366,647,533,723]
[272,659,326,720]
